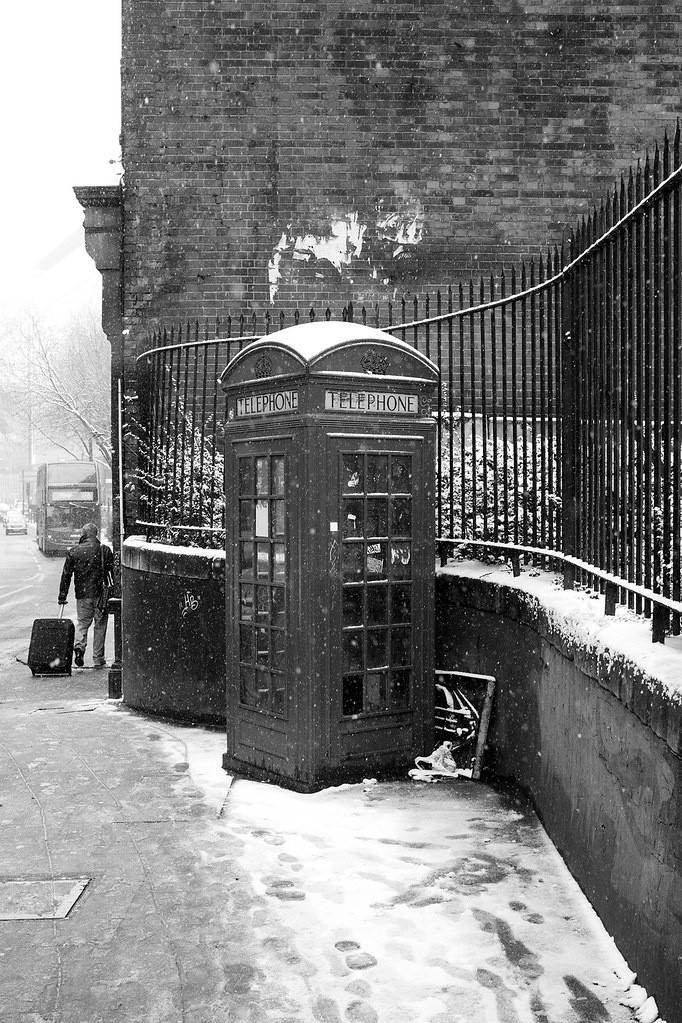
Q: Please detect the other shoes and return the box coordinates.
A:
[94,660,106,665]
[74,648,84,666]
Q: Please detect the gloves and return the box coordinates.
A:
[58,600,68,605]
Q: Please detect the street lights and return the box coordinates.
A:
[0,458,11,507]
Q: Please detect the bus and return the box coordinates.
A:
[36,460,102,558]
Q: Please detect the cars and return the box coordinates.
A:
[2,510,28,535]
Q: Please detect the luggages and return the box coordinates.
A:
[27,604,75,676]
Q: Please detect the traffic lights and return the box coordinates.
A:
[26,482,29,496]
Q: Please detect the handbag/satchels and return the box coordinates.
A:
[96,582,114,614]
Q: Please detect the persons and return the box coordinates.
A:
[57,522,116,668]
